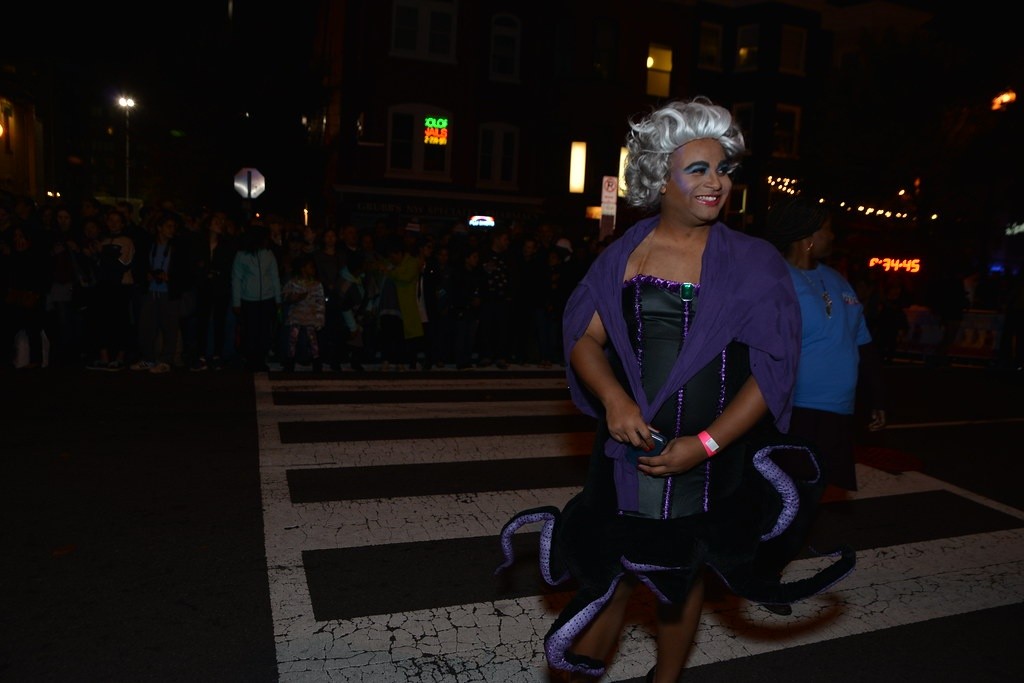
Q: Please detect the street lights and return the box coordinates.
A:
[111,90,143,200]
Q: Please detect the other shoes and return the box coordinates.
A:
[190,356,209,371]
[86,360,108,370]
[151,362,170,373]
[212,356,223,370]
[496,357,507,369]
[104,360,126,371]
[380,362,389,371]
[130,360,152,370]
[312,357,324,376]
[768,603,791,615]
[284,358,294,374]
[409,356,417,371]
[396,363,404,373]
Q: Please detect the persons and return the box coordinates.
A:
[0,191,606,373]
[490,101,801,683]
[841,265,895,370]
[772,201,887,490]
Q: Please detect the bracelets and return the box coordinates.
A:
[697,431,720,457]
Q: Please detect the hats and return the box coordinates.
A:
[556,238,574,254]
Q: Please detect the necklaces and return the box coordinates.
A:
[802,270,832,320]
